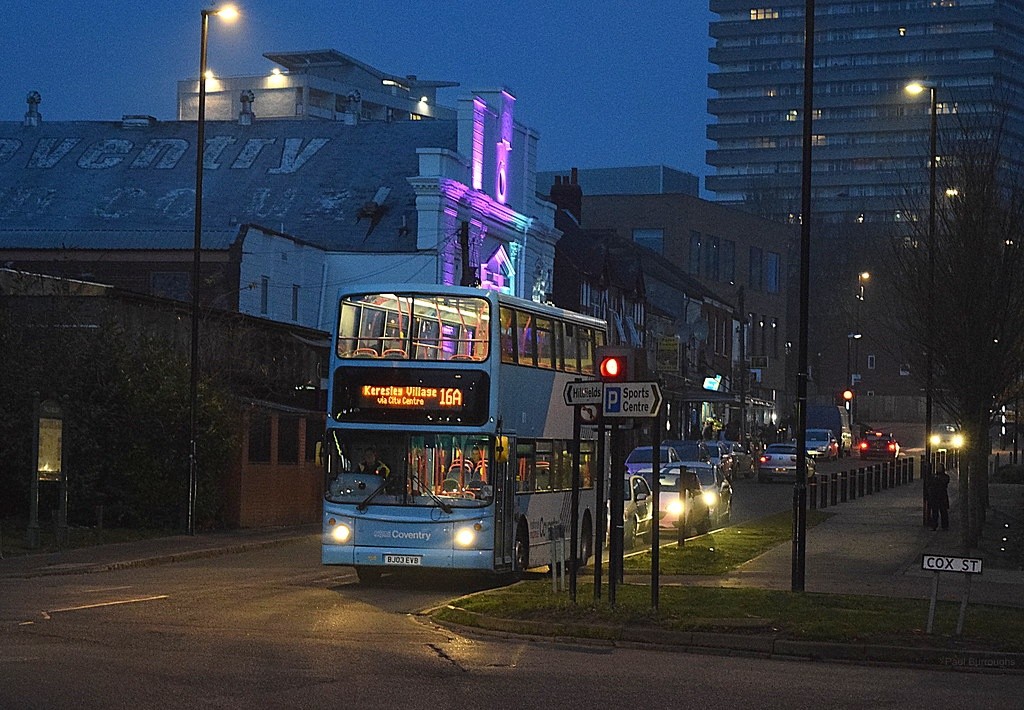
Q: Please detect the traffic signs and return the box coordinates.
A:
[562,380,604,406]
[601,381,664,417]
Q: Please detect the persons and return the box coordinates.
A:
[354,445,390,479]
[926,462,950,531]
[691,420,740,441]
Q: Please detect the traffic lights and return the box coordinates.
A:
[598,355,626,383]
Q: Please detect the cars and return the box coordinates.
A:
[931,424,964,448]
[660,438,713,463]
[757,443,816,484]
[606,475,651,550]
[623,445,682,493]
[805,428,838,461]
[702,439,755,481]
[634,460,735,539]
[859,430,898,462]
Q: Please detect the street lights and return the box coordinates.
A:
[846,333,862,431]
[904,81,938,530]
[185,5,243,538]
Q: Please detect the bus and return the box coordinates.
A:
[313,281,614,588]
[806,404,854,459]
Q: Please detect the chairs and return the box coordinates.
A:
[440,460,585,498]
[355,347,591,374]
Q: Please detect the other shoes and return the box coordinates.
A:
[930,526,940,531]
[943,527,950,532]
